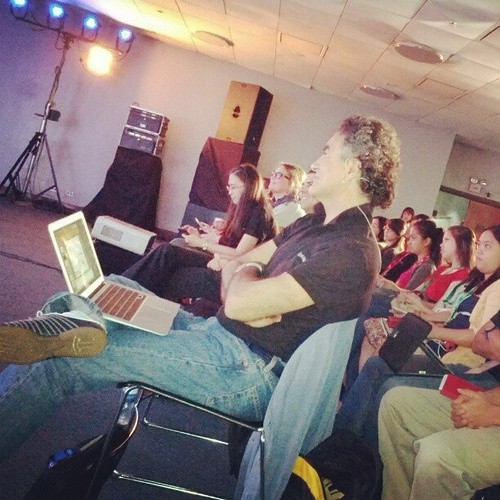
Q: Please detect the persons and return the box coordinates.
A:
[359,207,500,500]
[0,116,400,500]
[121,162,307,320]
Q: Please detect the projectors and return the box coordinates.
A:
[90,215,158,257]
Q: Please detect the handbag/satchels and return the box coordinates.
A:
[22,418,133,500]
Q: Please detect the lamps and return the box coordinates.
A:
[9,0,32,17]
[47,0,69,28]
[115,27,135,52]
[79,12,101,39]
[468,177,487,194]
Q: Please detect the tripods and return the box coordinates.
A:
[0,47,67,210]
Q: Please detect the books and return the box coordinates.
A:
[387,315,402,329]
[439,373,485,400]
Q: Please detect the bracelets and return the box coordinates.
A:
[235,262,263,275]
[202,241,208,251]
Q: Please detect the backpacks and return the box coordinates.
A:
[279,428,384,500]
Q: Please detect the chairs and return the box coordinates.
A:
[86,310,363,500]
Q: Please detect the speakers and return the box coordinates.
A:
[216,81,274,149]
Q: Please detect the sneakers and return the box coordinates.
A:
[0,310,108,365]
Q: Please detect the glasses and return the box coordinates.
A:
[226,185,245,190]
[271,172,292,181]
[383,226,392,232]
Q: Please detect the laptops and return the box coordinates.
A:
[46,211,182,337]
[378,312,452,380]
